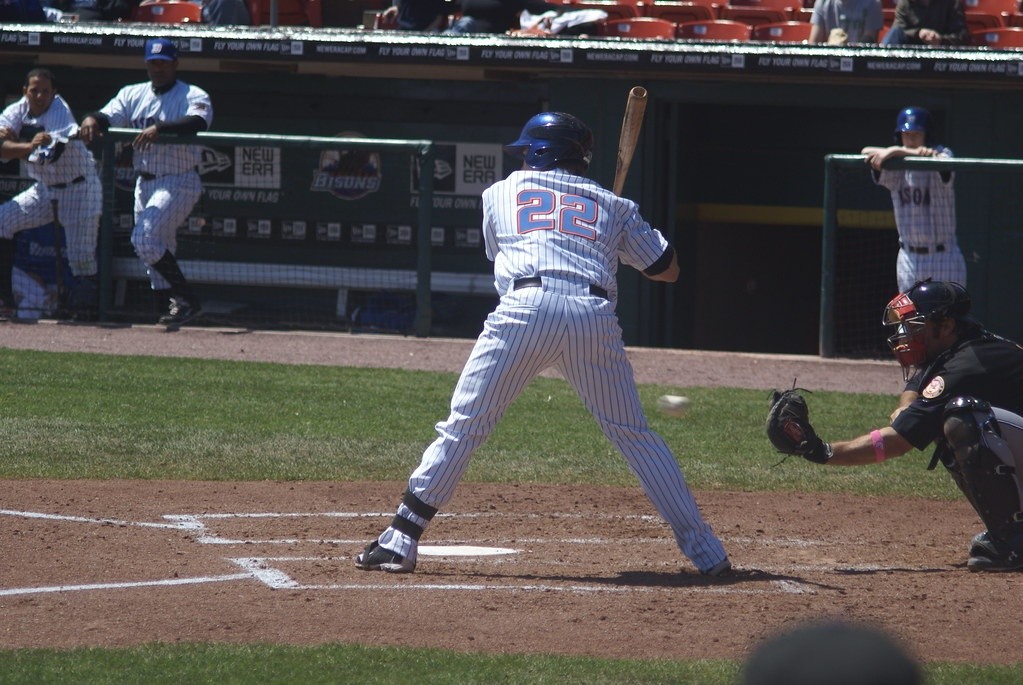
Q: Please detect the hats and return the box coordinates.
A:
[145,38,178,62]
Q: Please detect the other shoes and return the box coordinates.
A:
[67,306,98,323]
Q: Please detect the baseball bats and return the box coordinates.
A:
[49,197,67,309]
[612,86,649,196]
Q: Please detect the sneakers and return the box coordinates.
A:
[355,546,414,573]
[968,531,1023,571]
[160,296,202,325]
[702,557,731,577]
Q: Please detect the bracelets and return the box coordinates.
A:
[870,429,885,462]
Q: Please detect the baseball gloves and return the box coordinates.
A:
[765,391,833,465]
[28,123,79,165]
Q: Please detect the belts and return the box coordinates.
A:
[899,240,944,254]
[140,172,156,180]
[51,175,85,190]
[513,277,610,302]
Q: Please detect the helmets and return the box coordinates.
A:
[901,281,981,330]
[895,106,935,147]
[502,112,594,177]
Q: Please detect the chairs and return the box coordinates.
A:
[135,0,204,26]
[545,0,1023,50]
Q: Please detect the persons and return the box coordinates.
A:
[353,111,731,576]
[0,0,251,26]
[764,279,1023,572]
[378,0,554,33]
[80,38,213,325]
[882,0,969,45]
[862,106,967,332]
[0,68,101,321]
[808,0,884,44]
